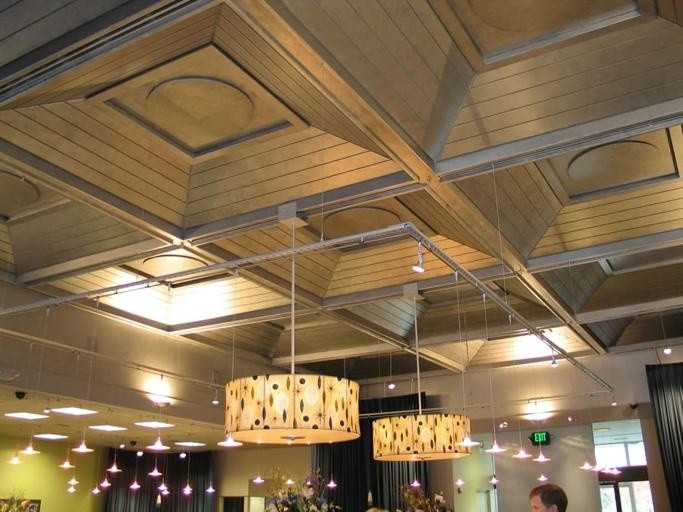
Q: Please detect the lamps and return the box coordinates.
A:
[371,296,481,466]
[479,290,622,475]
[408,243,430,274]
[222,220,363,450]
[7,294,244,499]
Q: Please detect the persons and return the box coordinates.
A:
[528,483,568,512]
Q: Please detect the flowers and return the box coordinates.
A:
[261,466,342,511]
[396,481,457,512]
